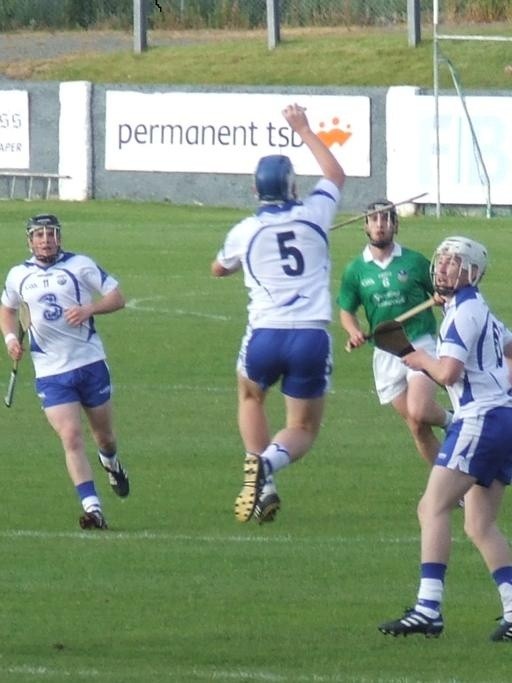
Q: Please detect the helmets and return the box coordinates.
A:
[255,155,300,208]
[27,215,62,263]
[429,236,487,295]
[365,199,398,247]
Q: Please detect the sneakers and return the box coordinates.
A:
[97,451,129,496]
[491,615,512,640]
[378,606,443,638]
[79,511,108,530]
[234,452,281,523]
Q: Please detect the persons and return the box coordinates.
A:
[338,199,455,469]
[377,234,512,638]
[0,212,129,532]
[208,102,349,523]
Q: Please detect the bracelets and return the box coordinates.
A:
[2,329,19,342]
